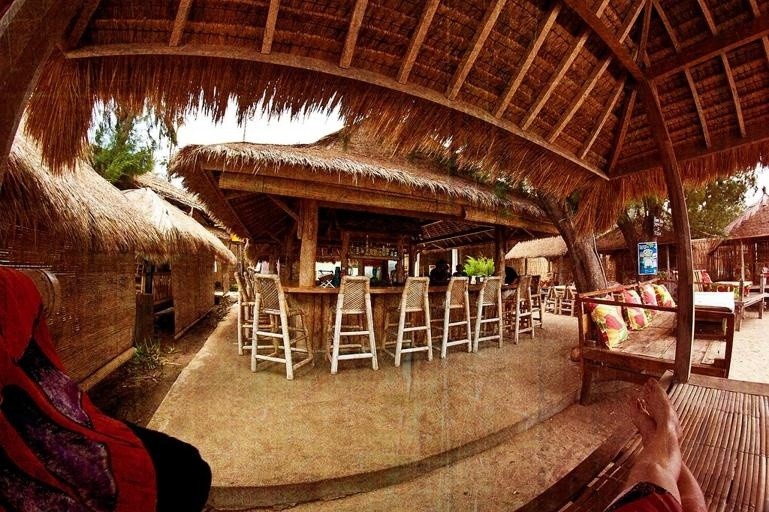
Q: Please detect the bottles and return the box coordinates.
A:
[733,285,739,299]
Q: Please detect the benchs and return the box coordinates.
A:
[570,277,739,407]
[673,269,766,330]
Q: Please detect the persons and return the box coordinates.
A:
[391,259,519,318]
[731,262,769,286]
[596,373,709,512]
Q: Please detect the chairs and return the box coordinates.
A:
[544,281,578,317]
[517,368,769,512]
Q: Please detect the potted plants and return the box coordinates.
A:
[465,255,495,286]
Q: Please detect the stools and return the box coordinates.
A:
[531,274,540,314]
[501,276,534,344]
[460,275,503,352]
[235,271,281,356]
[250,273,315,379]
[424,277,472,359]
[324,276,378,374]
[381,277,432,366]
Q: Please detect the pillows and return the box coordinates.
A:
[583,280,676,351]
[699,269,714,290]
